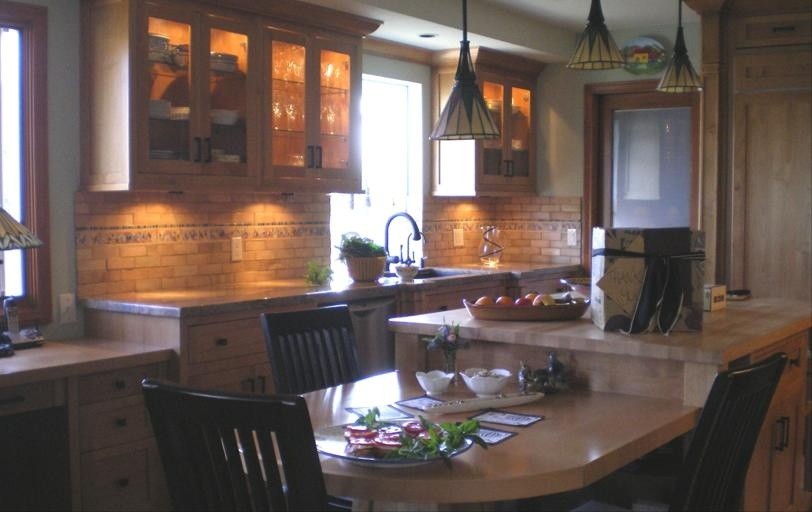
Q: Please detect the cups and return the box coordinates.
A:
[147,32,170,63]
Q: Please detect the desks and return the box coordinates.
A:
[0,337,178,512]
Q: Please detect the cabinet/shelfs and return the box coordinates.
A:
[721,325,812,512]
[399,277,584,315]
[83,304,319,403]
[430,66,540,200]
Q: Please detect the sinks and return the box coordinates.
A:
[385,264,482,279]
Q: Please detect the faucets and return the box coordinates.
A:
[383,209,425,266]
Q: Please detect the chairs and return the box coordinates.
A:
[140,380,352,512]
[260,304,362,397]
[554,353,788,512]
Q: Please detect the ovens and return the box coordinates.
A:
[321,297,397,379]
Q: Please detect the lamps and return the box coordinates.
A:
[656,0,705,95]
[566,0,626,70]
[429,0,501,141]
[0,207,46,356]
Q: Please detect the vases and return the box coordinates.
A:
[443,347,457,382]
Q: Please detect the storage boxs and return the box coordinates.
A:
[591,225,705,334]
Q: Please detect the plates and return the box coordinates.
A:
[150,97,242,163]
[484,96,521,116]
[426,391,545,414]
[461,293,591,320]
[313,418,477,472]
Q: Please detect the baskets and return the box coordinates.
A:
[345,255,387,282]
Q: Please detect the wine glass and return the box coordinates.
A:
[273,49,348,169]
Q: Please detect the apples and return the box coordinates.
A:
[515,297,532,306]
[532,294,555,306]
[525,293,536,302]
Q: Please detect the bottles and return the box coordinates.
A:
[478,226,503,267]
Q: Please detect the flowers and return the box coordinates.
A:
[421,324,469,350]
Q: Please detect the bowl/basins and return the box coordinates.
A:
[170,43,239,72]
[459,366,514,401]
[416,369,456,397]
[395,265,419,281]
[560,277,591,299]
